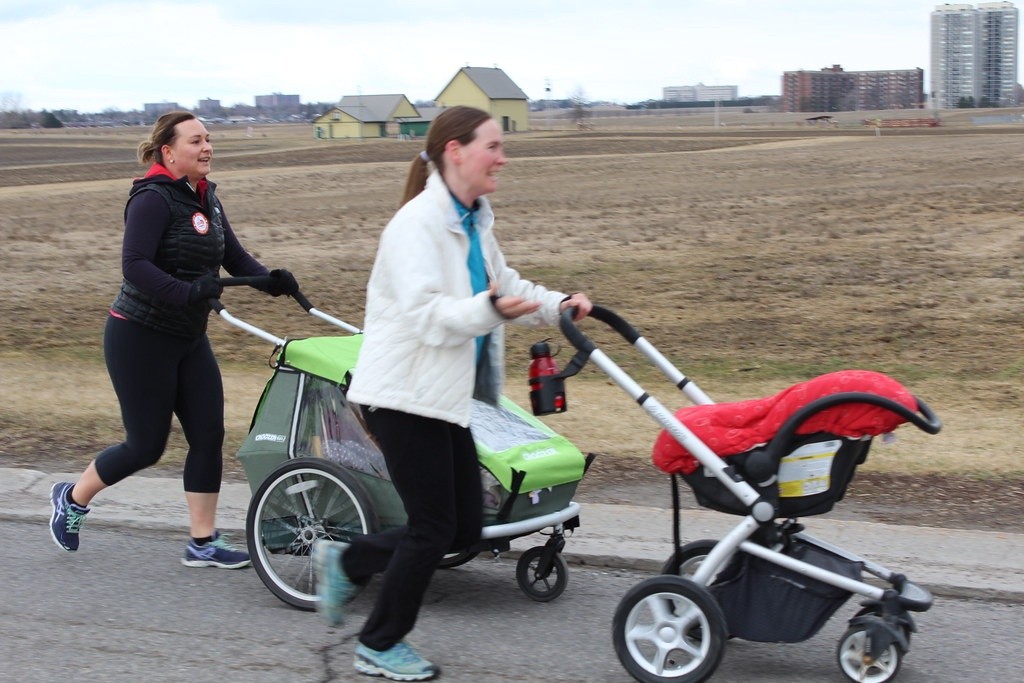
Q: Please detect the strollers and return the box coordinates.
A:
[210,275,596,612]
[529,306,943,683]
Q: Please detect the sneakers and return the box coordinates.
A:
[50,481,93,552]
[353,635,439,681]
[314,538,363,628]
[181,527,251,569]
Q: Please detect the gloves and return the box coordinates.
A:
[268,266,299,297]
[188,276,222,308]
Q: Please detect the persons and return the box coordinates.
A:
[48,110,299,567]
[309,105,592,682]
